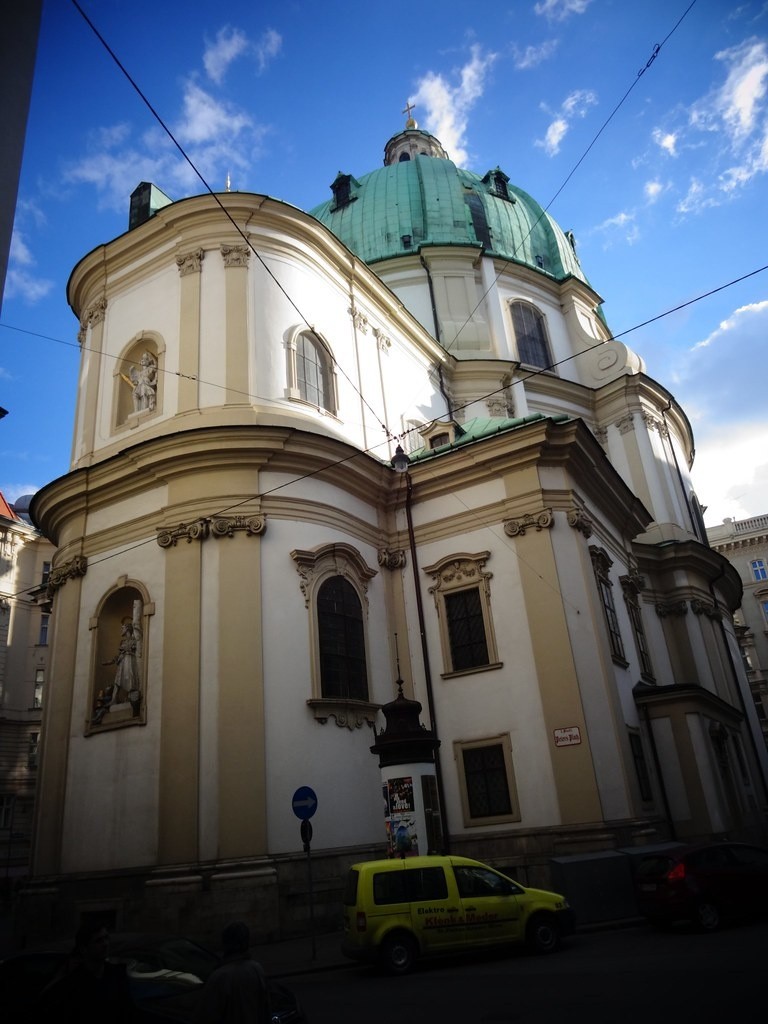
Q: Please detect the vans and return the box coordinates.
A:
[341,852,579,979]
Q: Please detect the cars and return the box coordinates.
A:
[1,931,312,1024]
[632,841,768,936]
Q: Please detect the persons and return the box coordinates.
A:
[130,354,156,412]
[190,921,273,1023]
[113,623,139,703]
